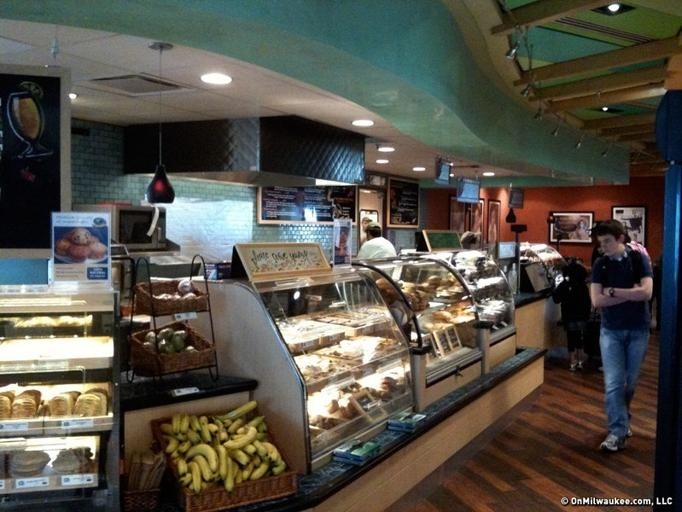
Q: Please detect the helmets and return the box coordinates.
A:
[135,280,208,314]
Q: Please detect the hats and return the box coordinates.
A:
[363,217,372,222]
[596,219,631,243]
[461,232,481,243]
[365,223,382,232]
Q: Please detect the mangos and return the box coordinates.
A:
[143,327,199,357]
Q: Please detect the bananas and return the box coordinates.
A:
[159,399,288,494]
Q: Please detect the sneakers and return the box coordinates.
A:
[569,360,578,371]
[627,422,633,437]
[600,434,624,451]
[578,360,584,368]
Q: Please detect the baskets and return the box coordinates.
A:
[151,409,298,512]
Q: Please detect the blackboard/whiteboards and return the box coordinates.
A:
[386,176,420,228]
[256,188,358,225]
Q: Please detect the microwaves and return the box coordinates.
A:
[72,202,167,250]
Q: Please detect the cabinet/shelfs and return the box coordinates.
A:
[129,252,218,385]
[0,284,126,512]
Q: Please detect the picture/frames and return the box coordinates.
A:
[446,195,502,248]
[610,204,649,249]
[548,211,595,245]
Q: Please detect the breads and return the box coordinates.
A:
[0,446,93,480]
[55,228,105,263]
[0,388,111,419]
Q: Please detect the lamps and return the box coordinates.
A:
[50,23,62,62]
[504,187,518,222]
[142,38,181,206]
[606,0,621,15]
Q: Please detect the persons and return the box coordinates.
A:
[551,220,653,372]
[488,209,497,241]
[471,206,481,232]
[356,223,397,260]
[451,200,464,233]
[460,232,479,250]
[591,219,653,451]
[360,217,373,242]
